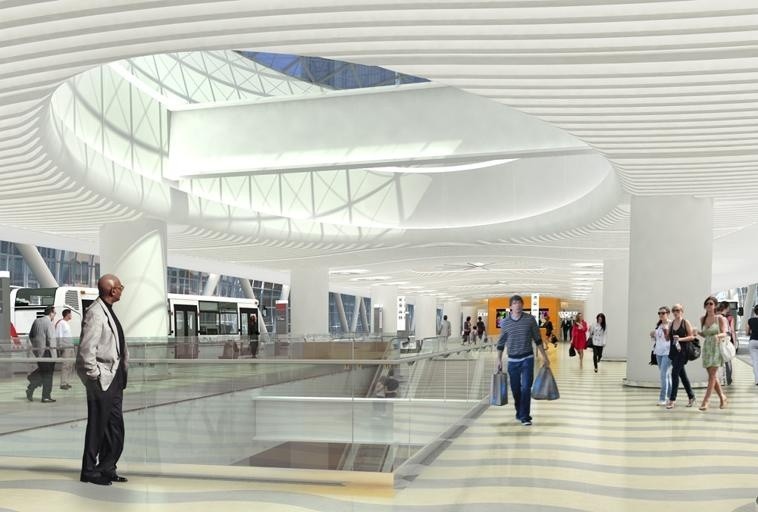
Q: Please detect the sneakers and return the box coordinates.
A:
[516,414,532,425]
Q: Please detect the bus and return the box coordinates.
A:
[715,298,744,333]
[8,286,268,361]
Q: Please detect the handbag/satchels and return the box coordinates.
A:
[489,369,509,405]
[719,336,736,360]
[685,338,701,360]
[649,350,658,365]
[569,343,576,356]
[587,337,593,348]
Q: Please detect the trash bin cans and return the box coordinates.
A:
[278,339,290,356]
[218,340,240,359]
[175,343,200,359]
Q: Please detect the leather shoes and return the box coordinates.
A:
[81,468,128,486]
[26,388,34,401]
[41,395,57,402]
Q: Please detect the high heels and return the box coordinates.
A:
[656,396,729,409]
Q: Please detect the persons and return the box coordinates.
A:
[461,316,472,346]
[470,326,479,346]
[55,308,73,390]
[588,313,608,373]
[374,376,398,397]
[539,315,558,351]
[661,302,697,409]
[438,315,451,344]
[26,305,58,403]
[697,295,727,412]
[648,305,673,406]
[568,317,576,340]
[374,369,400,397]
[571,313,588,368]
[476,316,487,342]
[72,274,130,486]
[248,315,260,360]
[496,295,551,426]
[560,317,570,341]
[745,304,758,387]
[716,300,737,387]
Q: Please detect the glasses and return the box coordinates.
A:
[658,312,669,315]
[706,303,715,306]
